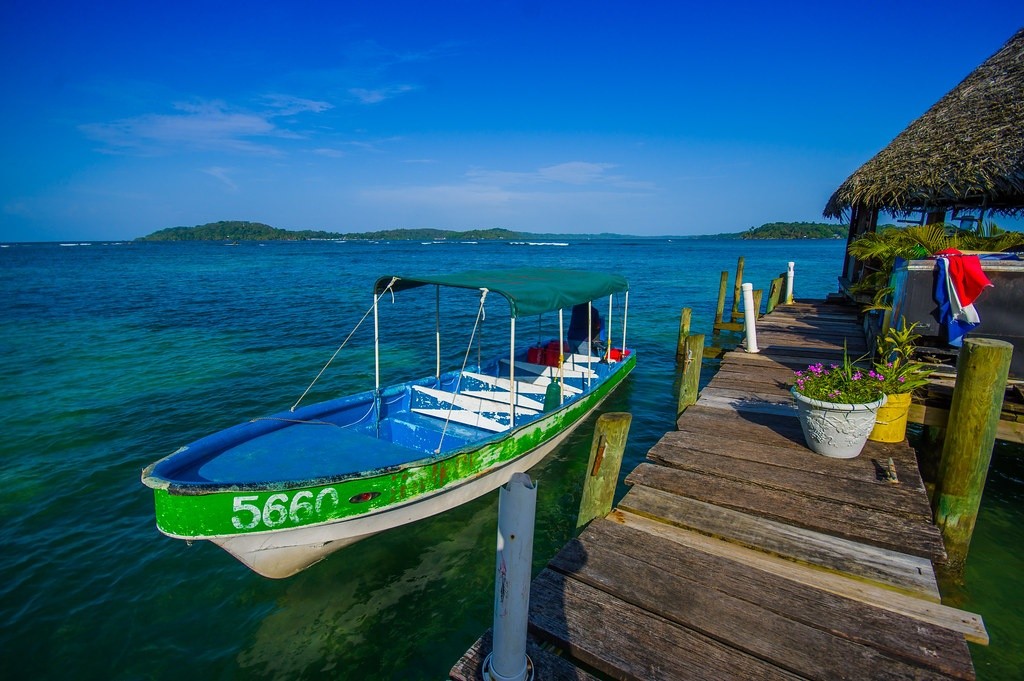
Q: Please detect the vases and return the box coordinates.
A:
[791,386,888,458]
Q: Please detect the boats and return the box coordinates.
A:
[138,264,637,581]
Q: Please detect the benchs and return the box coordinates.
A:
[387,349,612,452]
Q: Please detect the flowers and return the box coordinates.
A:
[783,336,905,405]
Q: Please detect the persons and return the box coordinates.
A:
[566,298,601,357]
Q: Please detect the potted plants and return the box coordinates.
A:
[866,317,934,443]
